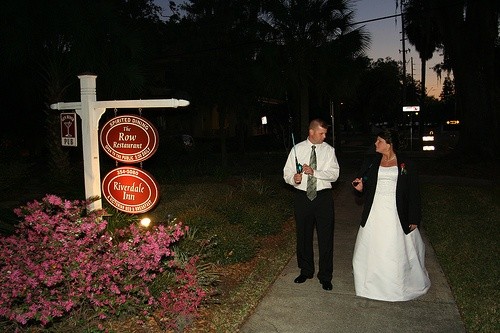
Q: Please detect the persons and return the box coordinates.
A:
[351,127,432,302]
[283,120,340,292]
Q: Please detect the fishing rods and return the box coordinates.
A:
[290,131,302,184]
[353,163,372,186]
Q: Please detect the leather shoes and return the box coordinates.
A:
[320,280,332,290]
[295,273,312,283]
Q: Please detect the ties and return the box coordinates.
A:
[307,147,317,200]
